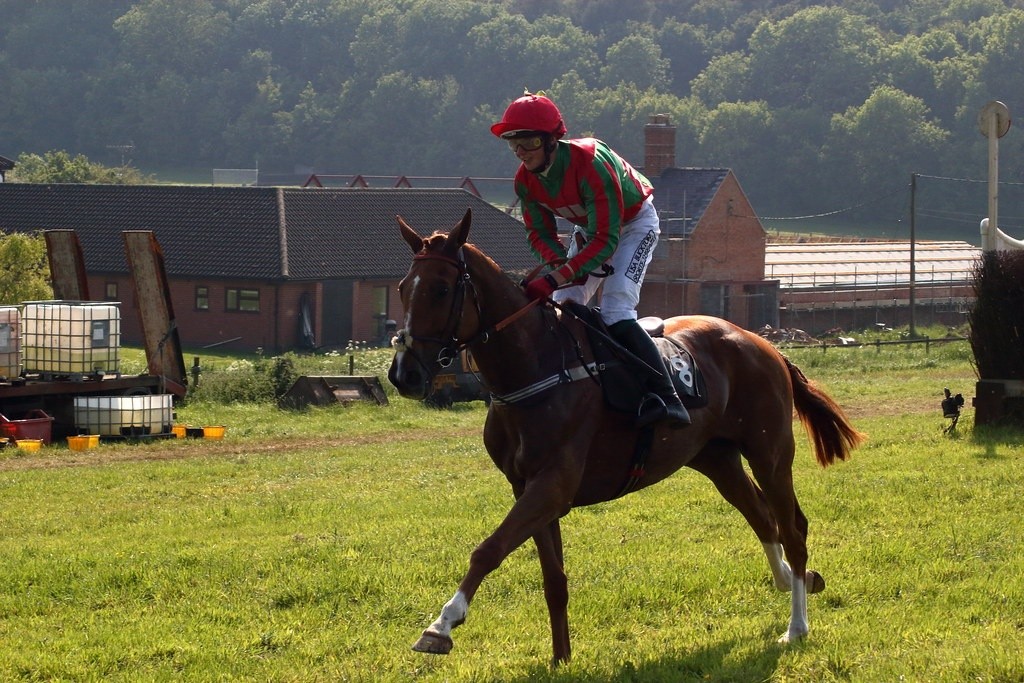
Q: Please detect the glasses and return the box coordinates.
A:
[507,133,545,152]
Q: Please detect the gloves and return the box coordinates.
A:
[527,273,558,297]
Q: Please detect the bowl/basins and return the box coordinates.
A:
[203,425,226,439]
[15,440,41,451]
[10,417,54,447]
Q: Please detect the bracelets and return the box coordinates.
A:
[565,263,574,275]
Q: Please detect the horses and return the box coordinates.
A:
[387,206,866,669]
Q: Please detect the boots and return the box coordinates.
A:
[608,318,692,428]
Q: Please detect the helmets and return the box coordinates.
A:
[491,94,567,137]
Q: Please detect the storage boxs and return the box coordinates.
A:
[0,416,55,446]
[171,424,187,439]
[14,438,45,452]
[66,434,100,451]
[202,425,226,440]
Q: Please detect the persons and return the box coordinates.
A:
[489,93,692,429]
